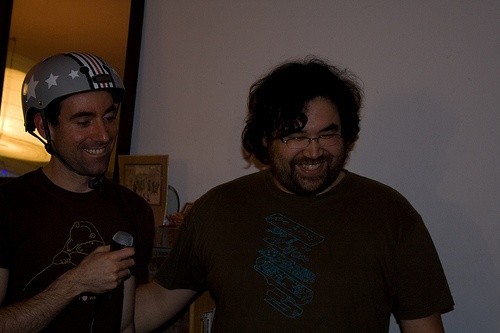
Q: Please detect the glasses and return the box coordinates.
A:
[279,134,343,149]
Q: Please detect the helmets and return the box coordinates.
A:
[20,52,126,134]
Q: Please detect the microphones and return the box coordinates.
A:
[93,231,134,318]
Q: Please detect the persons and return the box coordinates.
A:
[0,49,155,333]
[133,58,455,333]
